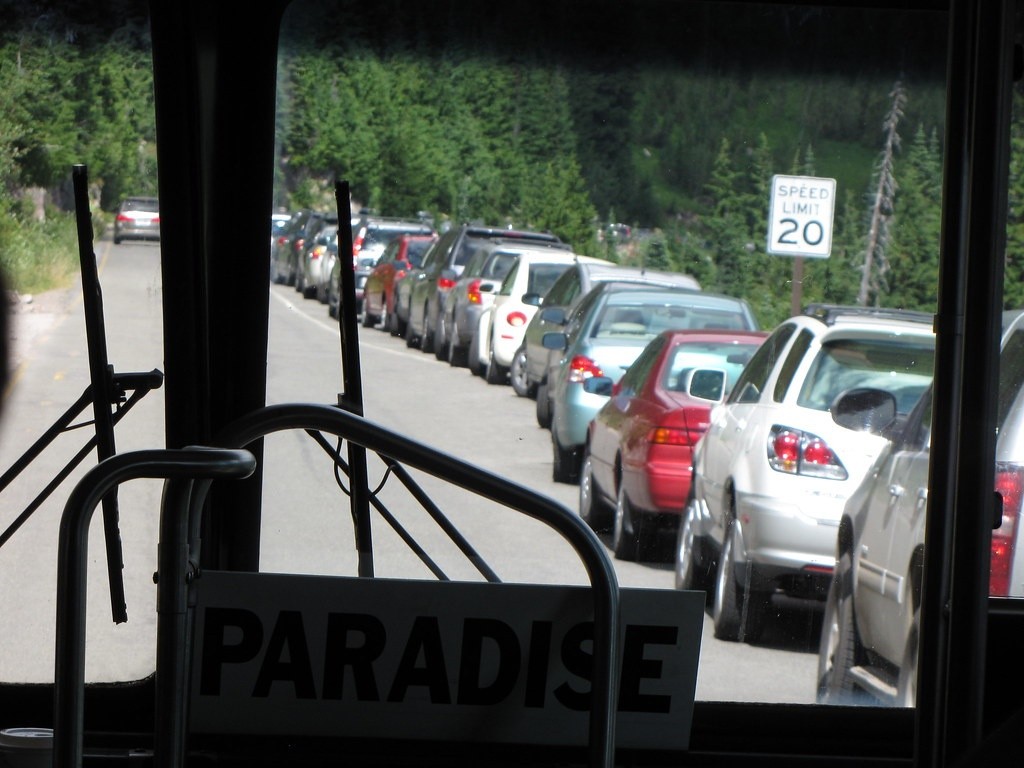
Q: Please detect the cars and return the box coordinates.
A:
[508,265,703,396]
[815,303,1024,711]
[572,329,771,561]
[538,278,763,484]
[113,196,161,244]
[605,223,631,246]
[270,209,576,367]
[475,249,618,383]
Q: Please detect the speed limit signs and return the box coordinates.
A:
[768,175,837,258]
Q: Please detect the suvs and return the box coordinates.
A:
[681,305,935,646]
